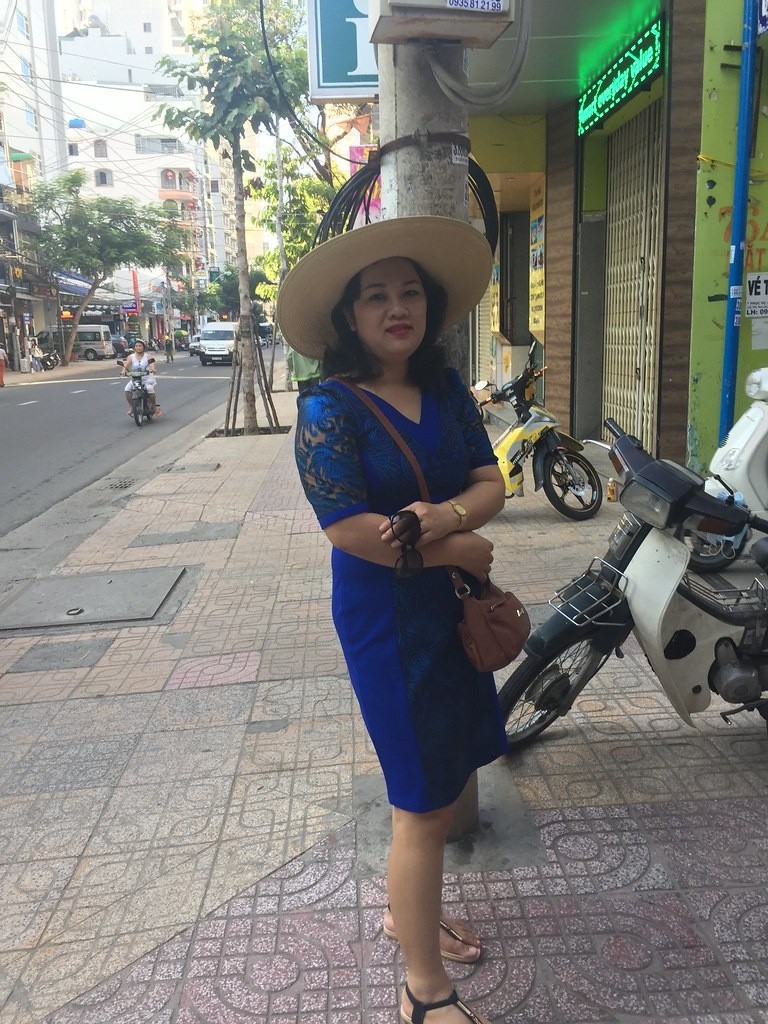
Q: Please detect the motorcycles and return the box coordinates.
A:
[116,358,160,426]
[493,370,768,753]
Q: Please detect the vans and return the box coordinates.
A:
[197,321,244,366]
[34,324,114,361]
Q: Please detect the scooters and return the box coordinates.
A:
[473,340,604,520]
[41,347,61,370]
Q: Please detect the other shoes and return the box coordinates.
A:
[127,407,133,416]
[153,405,161,418]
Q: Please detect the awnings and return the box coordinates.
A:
[1,290,43,303]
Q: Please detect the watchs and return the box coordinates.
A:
[448,500,467,532]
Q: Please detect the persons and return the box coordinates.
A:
[286,346,322,395]
[175,336,185,351]
[164,335,174,364]
[30,339,44,375]
[120,339,162,417]
[129,335,136,349]
[0,343,9,388]
[278,215,507,1024]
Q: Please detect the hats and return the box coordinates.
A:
[164,336,169,341]
[277,215,494,361]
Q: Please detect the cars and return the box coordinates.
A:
[254,334,282,348]
[189,335,202,357]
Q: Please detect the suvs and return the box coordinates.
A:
[107,335,129,359]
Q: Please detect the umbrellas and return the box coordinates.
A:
[174,329,188,337]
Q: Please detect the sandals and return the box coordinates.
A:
[398,984,481,1024]
[383,899,482,962]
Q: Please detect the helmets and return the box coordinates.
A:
[133,340,147,350]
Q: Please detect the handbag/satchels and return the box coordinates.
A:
[37,348,44,360]
[457,593,533,676]
